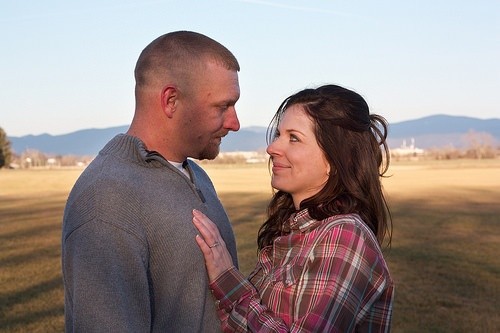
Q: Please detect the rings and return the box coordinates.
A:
[210,242,219,248]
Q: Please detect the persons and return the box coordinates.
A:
[193,85,396,333]
[61,30,239,333]
